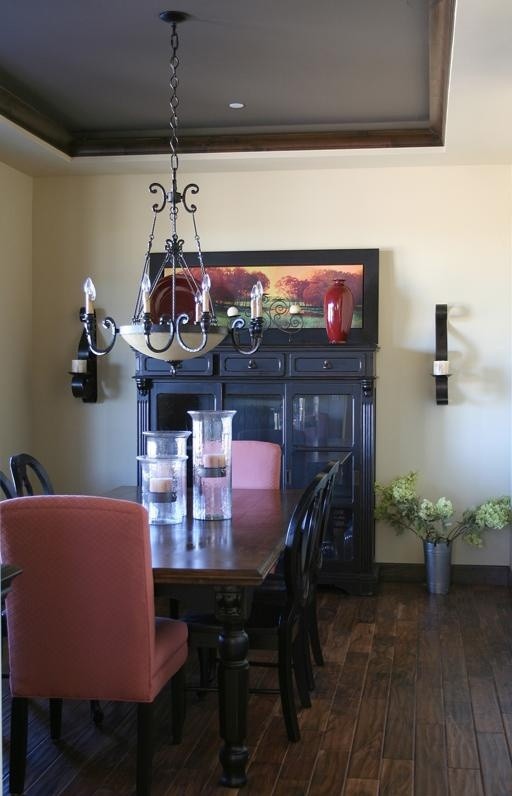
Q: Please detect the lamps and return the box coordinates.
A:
[81,11,304,375]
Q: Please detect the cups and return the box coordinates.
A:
[136,455,186,526]
[188,410,237,522]
[142,431,192,517]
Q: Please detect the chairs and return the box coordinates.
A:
[168,458,340,746]
[0,494,188,796]
[0,453,54,499]
[196,441,282,490]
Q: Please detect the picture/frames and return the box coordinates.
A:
[149,248,380,344]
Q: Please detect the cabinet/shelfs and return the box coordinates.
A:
[133,347,379,596]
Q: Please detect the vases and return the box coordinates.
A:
[423,539,452,595]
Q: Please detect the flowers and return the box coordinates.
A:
[375,469,512,551]
